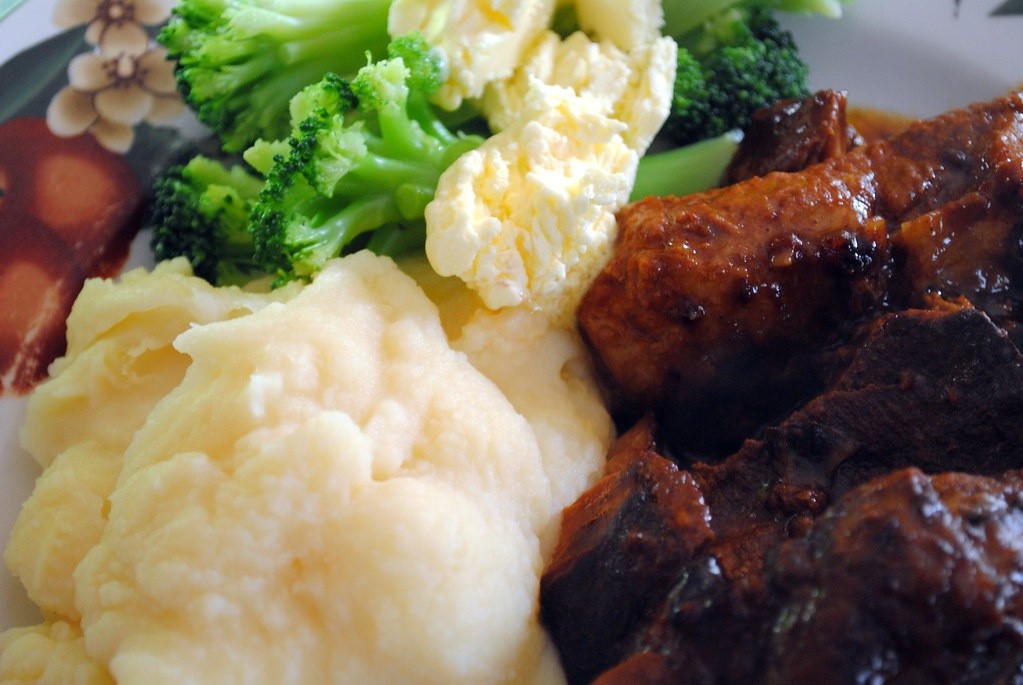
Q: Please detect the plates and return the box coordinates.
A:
[1,1,1023,628]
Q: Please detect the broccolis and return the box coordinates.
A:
[0,0,851,685]
